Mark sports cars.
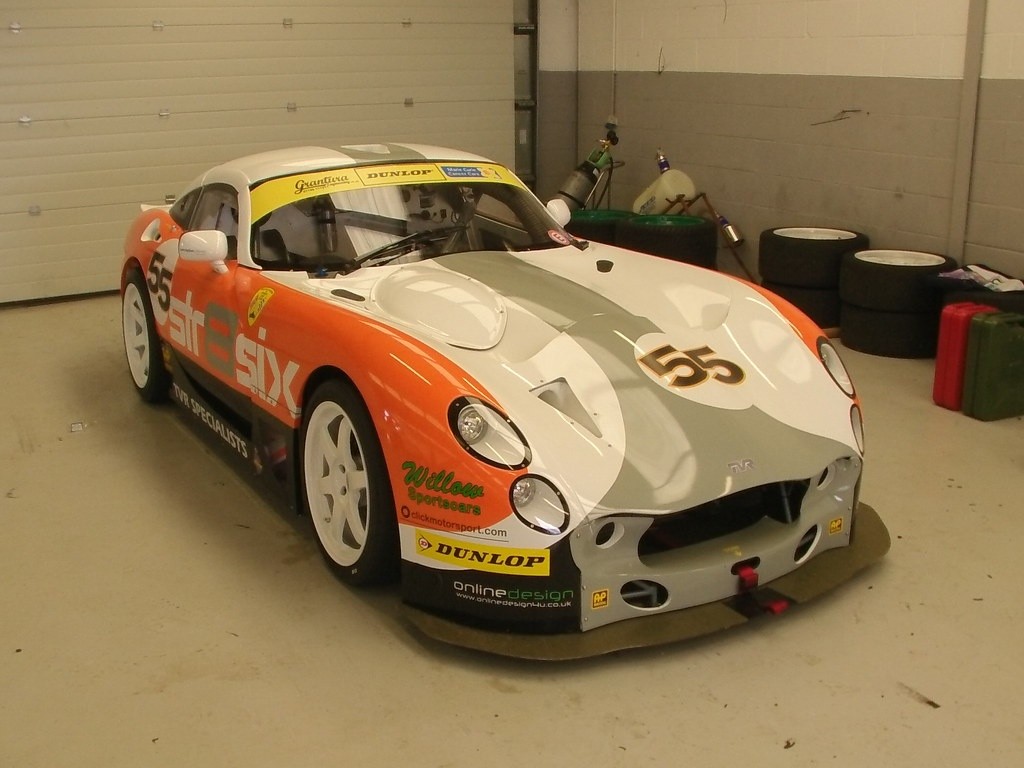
[121,143,892,661]
